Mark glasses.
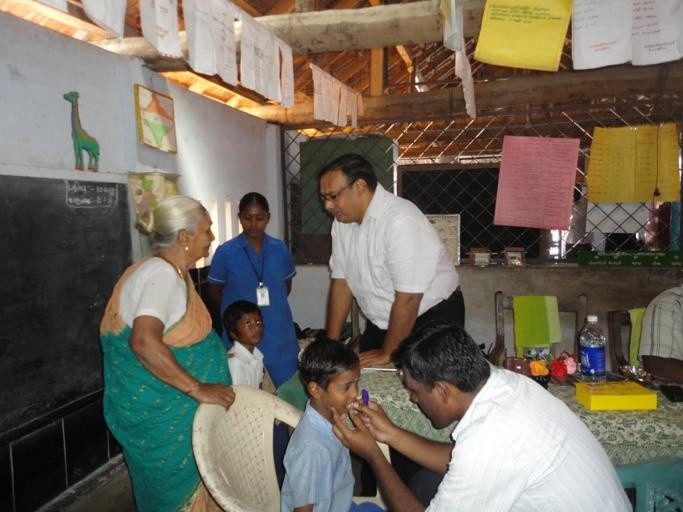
[320,181,354,203]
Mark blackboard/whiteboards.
[397,164,550,265]
[0,163,140,444]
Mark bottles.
[577,313,607,384]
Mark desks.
[272,368,682,511]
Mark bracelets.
[186,380,199,393]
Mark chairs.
[485,289,645,376]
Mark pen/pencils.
[362,390,370,417]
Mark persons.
[204,193,300,487]
[637,286,683,402]
[224,300,264,389]
[280,337,361,511]
[315,153,464,511]
[329,315,635,512]
[99,196,236,512]
